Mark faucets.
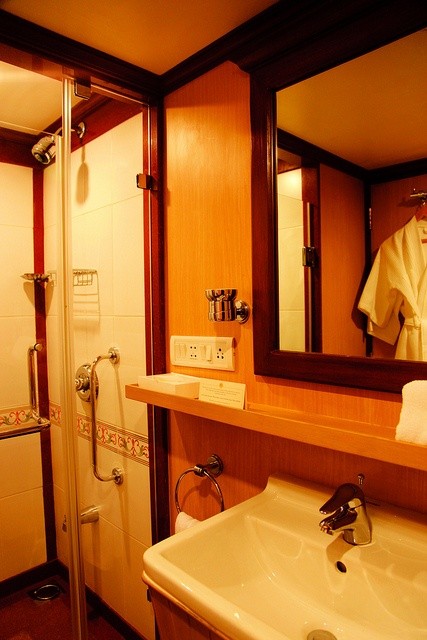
[320,482,372,545]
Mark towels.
[395,380,426,448]
[173,511,201,533]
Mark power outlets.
[169,335,236,373]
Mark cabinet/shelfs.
[143,586,228,639]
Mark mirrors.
[250,16,427,395]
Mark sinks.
[143,472,426,640]
